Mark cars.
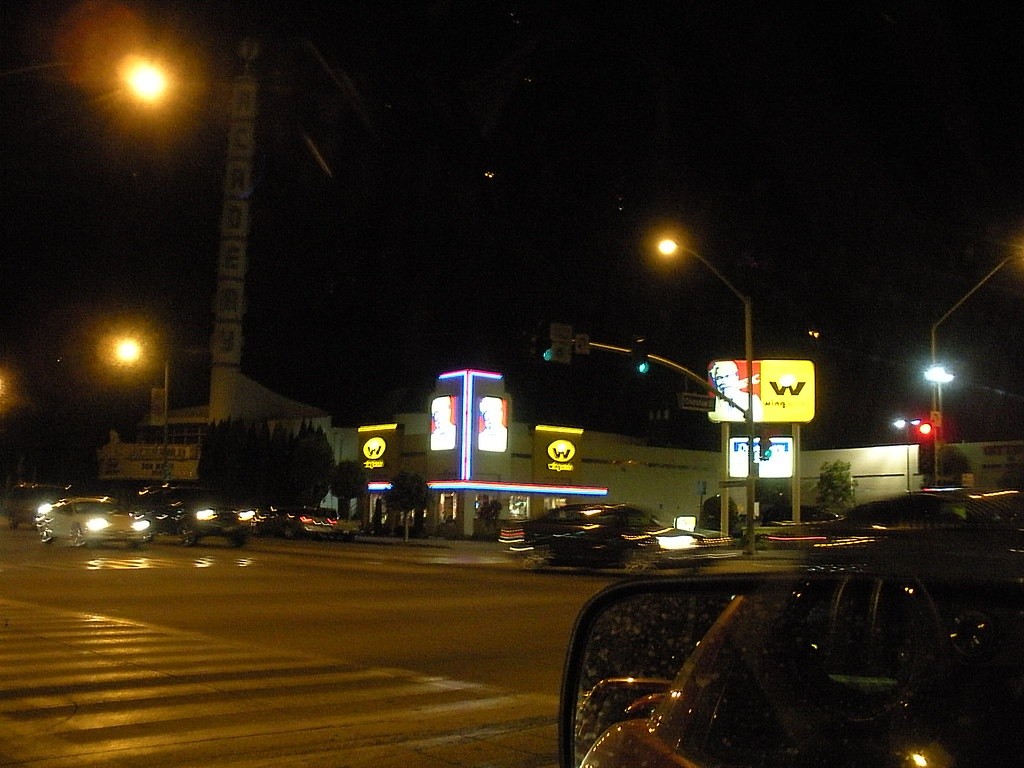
[250,500,361,542]
[576,679,673,767]
[36,498,156,550]
[520,503,728,573]
[808,486,1023,570]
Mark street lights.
[111,338,169,484]
[656,239,755,558]
[893,417,919,493]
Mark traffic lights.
[538,336,551,362]
[635,339,651,376]
[760,429,771,460]
[921,421,932,438]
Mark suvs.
[3,483,79,527]
[132,481,255,550]
